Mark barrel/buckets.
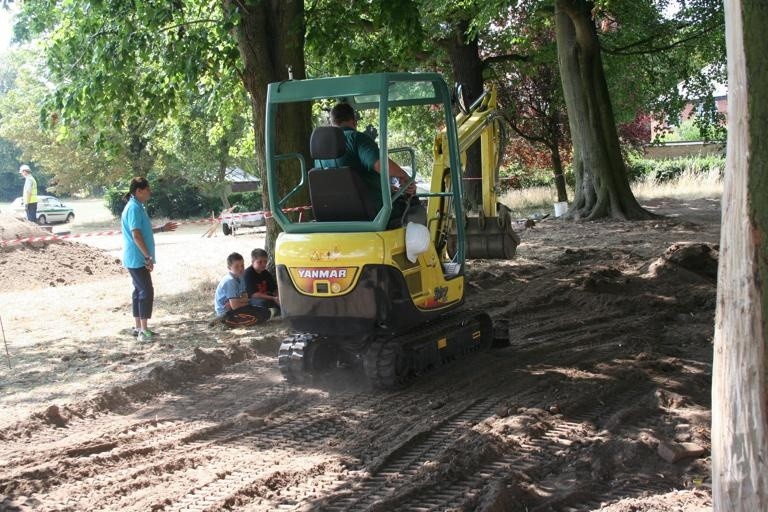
[553,202,568,217]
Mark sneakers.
[208,317,222,329]
[132,327,159,338]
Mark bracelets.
[161,226,165,232]
[145,255,152,261]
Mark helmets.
[405,221,430,263]
[19,165,30,174]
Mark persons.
[245,247,282,318]
[120,177,179,340]
[207,251,273,332]
[19,163,38,224]
[313,102,430,230]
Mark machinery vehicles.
[264,71,520,391]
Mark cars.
[9,194,75,226]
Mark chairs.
[308,126,408,231]
